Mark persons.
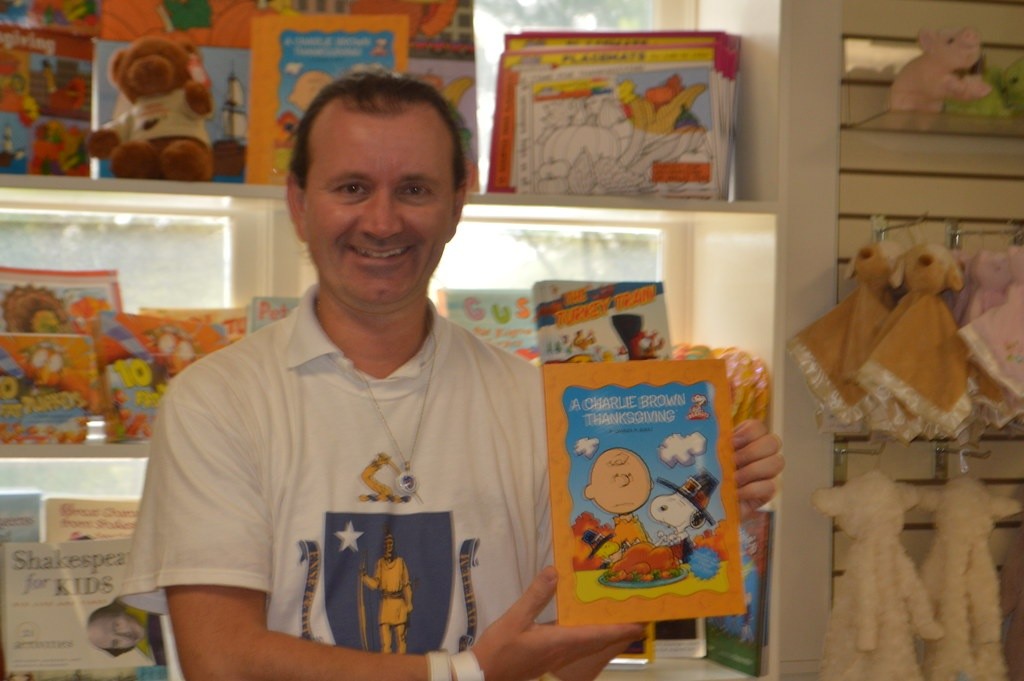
[120,70,786,680]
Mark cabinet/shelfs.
[2,0,1024,681]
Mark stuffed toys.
[915,474,1023,681]
[813,470,944,681]
[884,19,989,123]
[996,52,1023,112]
[939,57,1014,131]
[82,29,221,180]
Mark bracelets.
[423,635,455,680]
[453,650,487,680]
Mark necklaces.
[316,281,448,498]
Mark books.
[250,292,307,333]
[2,265,126,340]
[258,1,481,201]
[38,496,152,542]
[484,17,754,205]
[98,306,226,442]
[536,279,674,363]
[537,352,748,625]
[2,1,97,40]
[0,331,98,450]
[0,483,44,541]
[1,15,89,179]
[88,1,257,182]
[246,11,412,188]
[438,284,540,364]
[2,544,171,680]
[138,305,250,349]
